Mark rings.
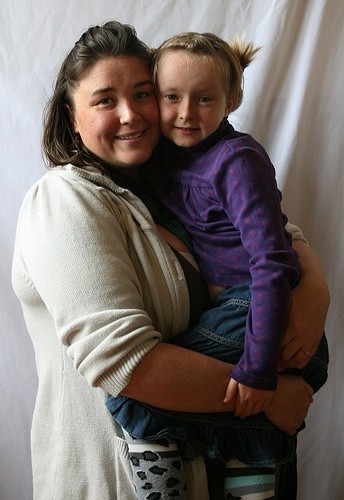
[299,348,312,358]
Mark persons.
[12,23,331,500]
[104,31,329,500]
